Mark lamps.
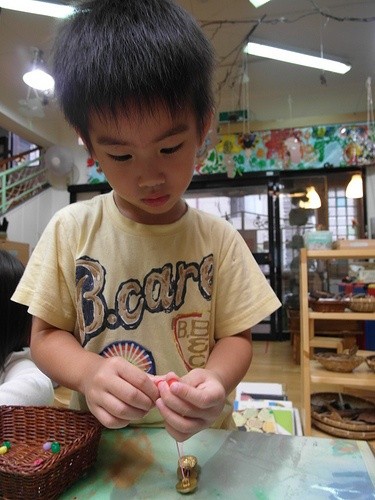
[0,0,75,20]
[242,16,352,75]
[22,51,55,91]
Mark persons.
[10,0,282,443]
[0,248,54,406]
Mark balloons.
[46,144,81,190]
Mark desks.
[57,428,375,500]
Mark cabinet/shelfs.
[298,248,375,454]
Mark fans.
[44,144,75,175]
